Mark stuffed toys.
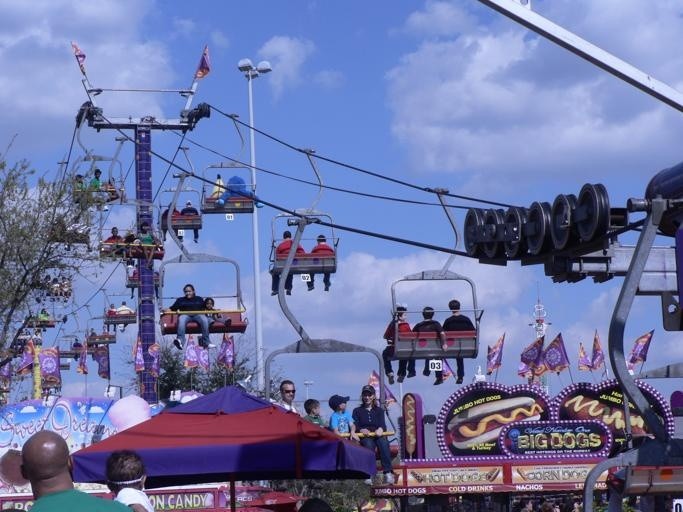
[211,174,263,209]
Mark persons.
[307,235,333,291]
[18,430,137,510]
[271,231,305,295]
[105,448,160,511]
[106,301,133,332]
[383,300,475,385]
[295,480,586,512]
[103,223,162,298]
[14,274,70,348]
[163,201,200,242]
[167,284,232,349]
[277,380,394,481]
[72,167,119,212]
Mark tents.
[68,387,375,488]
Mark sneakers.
[388,372,463,385]
[207,344,217,349]
[383,471,392,483]
[174,339,183,350]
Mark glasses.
[342,402,345,404]
[285,389,295,394]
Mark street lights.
[304,381,314,400]
[238,57,273,399]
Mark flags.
[368,371,396,406]
[4,333,235,388]
[191,45,214,79]
[483,329,654,385]
[70,34,91,77]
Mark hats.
[329,395,350,410]
[362,385,375,393]
